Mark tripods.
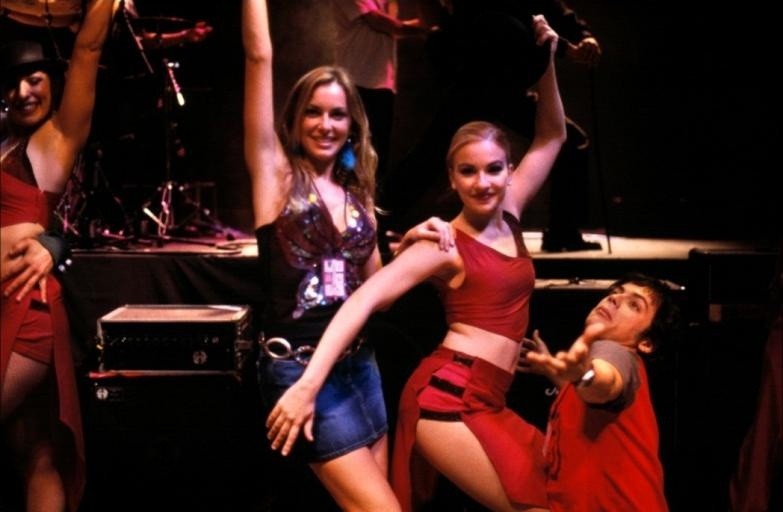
[59,47,238,250]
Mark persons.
[114,0,214,245]
[377,0,604,250]
[240,0,458,511]
[262,10,570,512]
[292,0,445,244]
[0,0,123,510]
[0,229,76,305]
[522,271,683,511]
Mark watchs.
[568,360,597,391]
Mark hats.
[0,38,68,77]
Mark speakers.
[505,277,676,501]
[688,248,783,512]
